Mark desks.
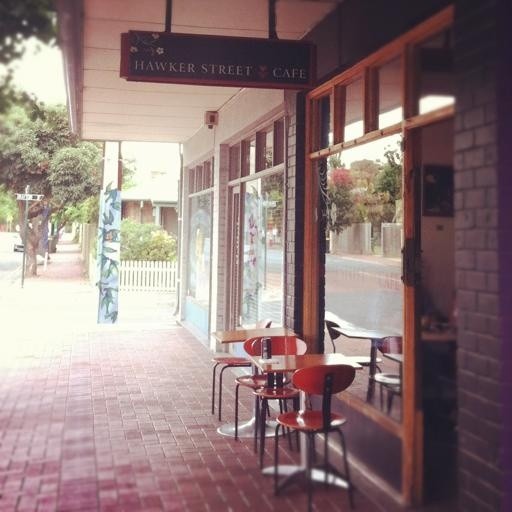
[213,329,301,438]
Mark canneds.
[260,338,272,359]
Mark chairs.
[325,320,402,415]
[234,334,360,512]
[213,321,272,423]
